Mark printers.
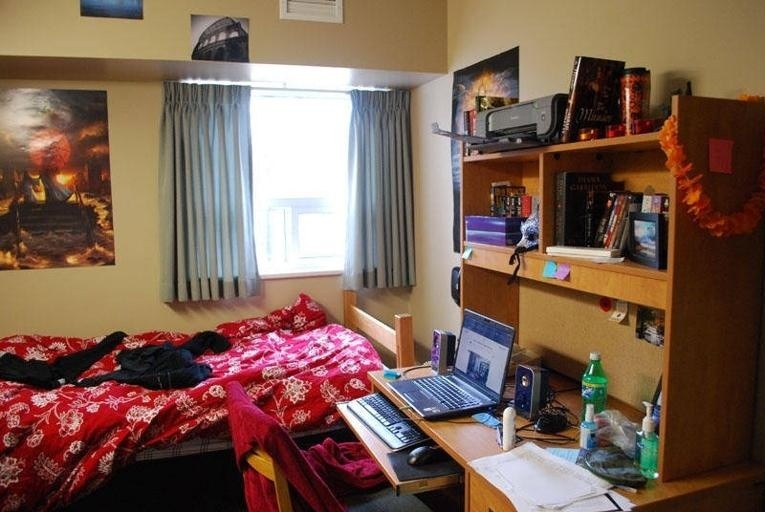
[432,94,567,153]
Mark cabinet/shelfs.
[335,94,764,510]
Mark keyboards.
[348,392,432,451]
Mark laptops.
[386,309,515,421]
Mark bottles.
[581,351,609,423]
[579,402,601,452]
[617,66,653,137]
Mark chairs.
[224,379,443,510]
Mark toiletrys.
[636,401,659,480]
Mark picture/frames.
[630,211,665,269]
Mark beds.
[1,288,414,512]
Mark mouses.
[408,445,437,466]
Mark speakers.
[432,329,456,375]
[515,365,550,420]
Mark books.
[490,180,532,218]
[546,172,670,257]
[559,56,625,143]
